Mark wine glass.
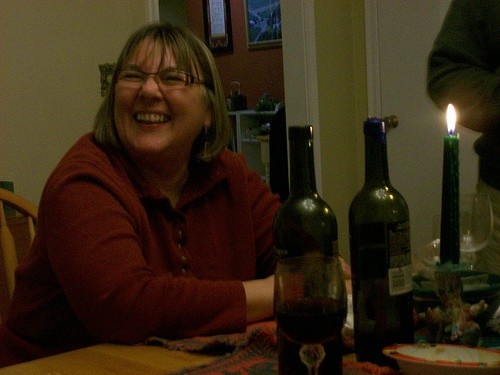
[459,193,493,269]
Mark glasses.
[116,67,211,90]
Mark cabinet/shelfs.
[229,102,283,172]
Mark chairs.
[0,186,38,296]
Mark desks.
[0,322,403,375]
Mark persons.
[0,22,351,370]
[425,0,500,276]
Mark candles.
[440,103,461,266]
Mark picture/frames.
[202,0,233,53]
[244,0,284,52]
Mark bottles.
[348,117,411,363]
[273,124,344,375]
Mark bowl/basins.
[381,341,500,375]
[413,266,500,334]
